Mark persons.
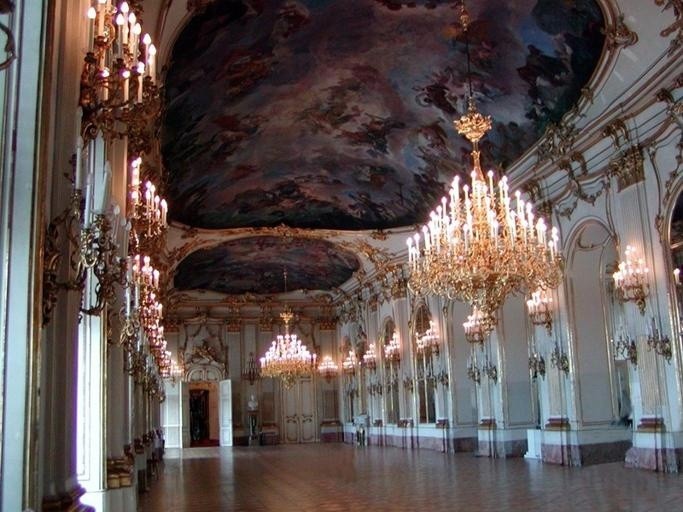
[248,394,258,435]
